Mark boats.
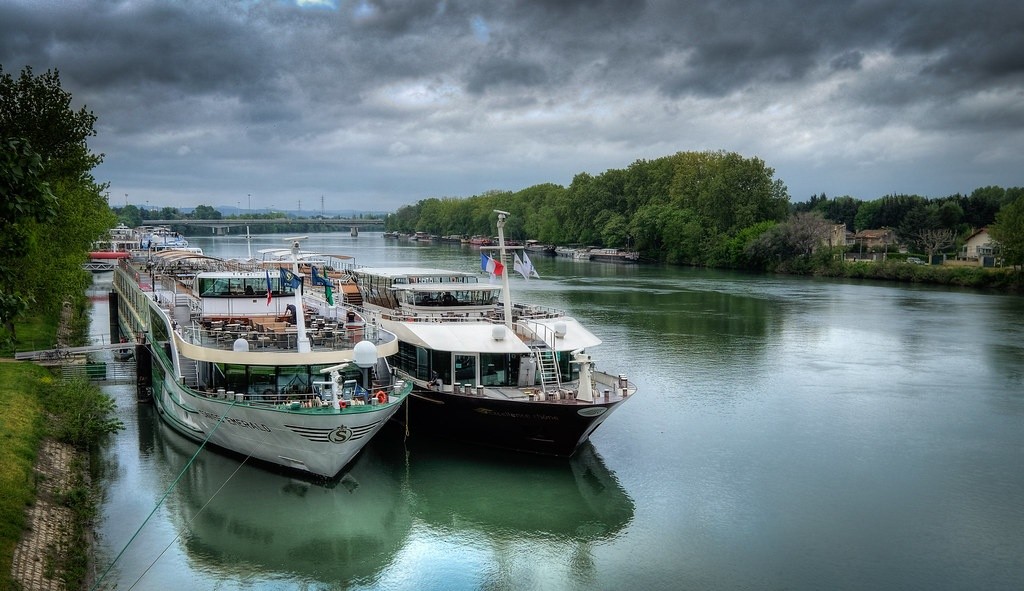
[553,244,590,262]
[591,246,632,260]
[86,223,204,266]
[524,238,550,253]
[353,204,637,470]
[468,237,491,245]
[410,231,432,243]
[382,232,400,239]
[113,245,413,479]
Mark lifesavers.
[375,390,386,404]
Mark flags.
[312,266,335,306]
[514,251,540,281]
[280,268,301,289]
[481,253,504,283]
[266,270,271,305]
[355,384,369,397]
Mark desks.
[147,261,209,286]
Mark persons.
[284,304,296,323]
[220,285,231,296]
[178,261,182,270]
[426,370,443,393]
[456,356,473,368]
[148,240,151,248]
[347,306,356,323]
[140,239,143,249]
[172,230,181,237]
[119,337,129,353]
[243,285,254,295]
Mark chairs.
[203,307,351,348]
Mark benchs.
[258,323,291,334]
[268,328,296,342]
[255,320,287,330]
[267,326,294,334]
[248,317,276,329]
